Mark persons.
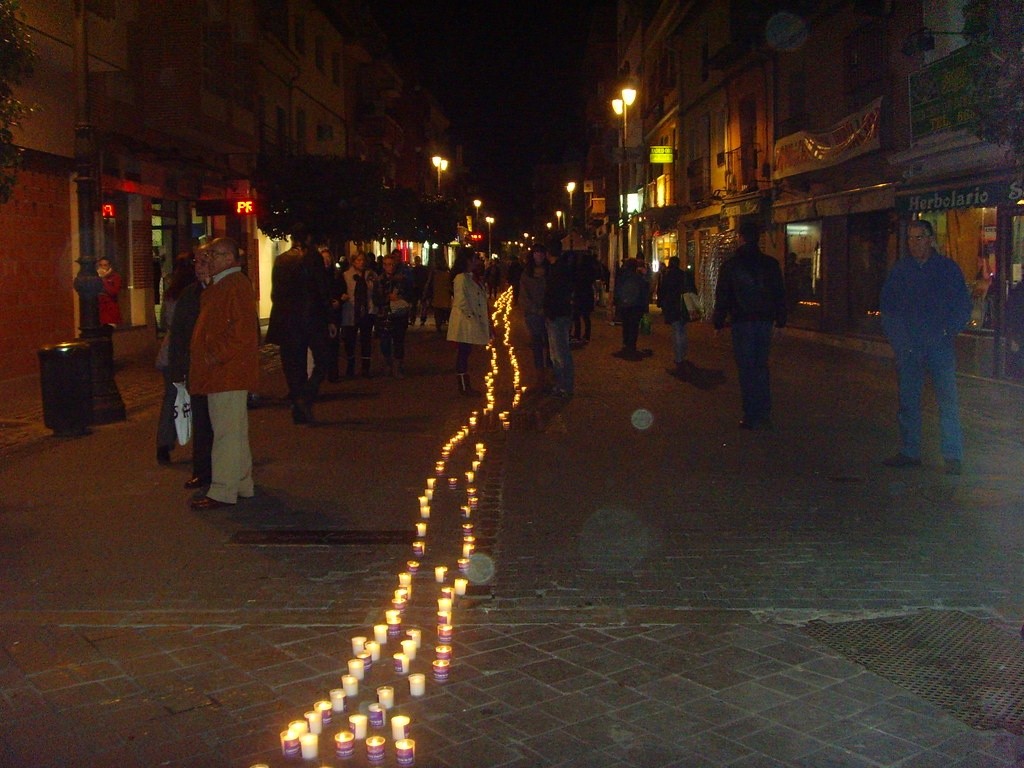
[256,221,338,425]
[878,218,973,477]
[710,221,789,432]
[294,237,699,405]
[151,236,277,513]
[1006,264,1024,380]
[784,252,803,310]
[96,256,123,357]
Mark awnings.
[629,177,1024,227]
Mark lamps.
[739,179,771,195]
[710,189,728,202]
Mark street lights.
[485,214,493,268]
[609,61,638,259]
[566,181,576,254]
[472,196,482,255]
[428,141,448,242]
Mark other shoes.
[883,452,920,468]
[944,460,961,476]
[192,497,234,510]
[186,475,205,488]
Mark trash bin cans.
[37,342,97,437]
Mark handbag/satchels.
[247,343,289,398]
[172,377,193,447]
[683,291,703,322]
[390,286,413,314]
[639,317,652,335]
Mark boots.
[457,372,481,399]
